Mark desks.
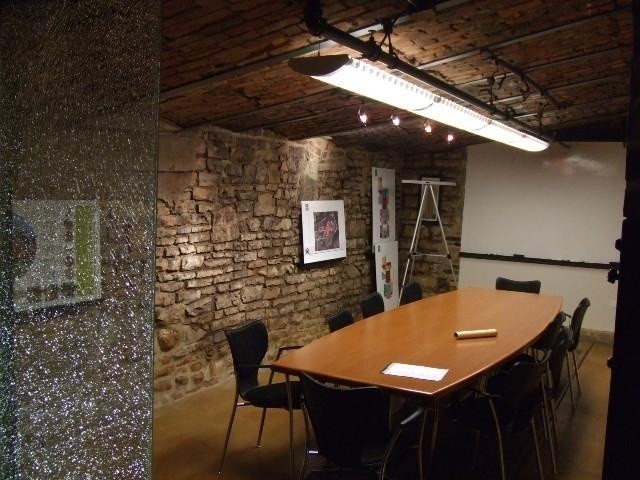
[270,286,563,477]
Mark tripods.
[396,185,457,306]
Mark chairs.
[298,372,429,480]
[358,293,385,320]
[218,321,306,475]
[326,310,353,333]
[430,277,591,480]
[398,282,422,306]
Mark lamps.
[288,2,554,154]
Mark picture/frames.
[299,199,348,264]
